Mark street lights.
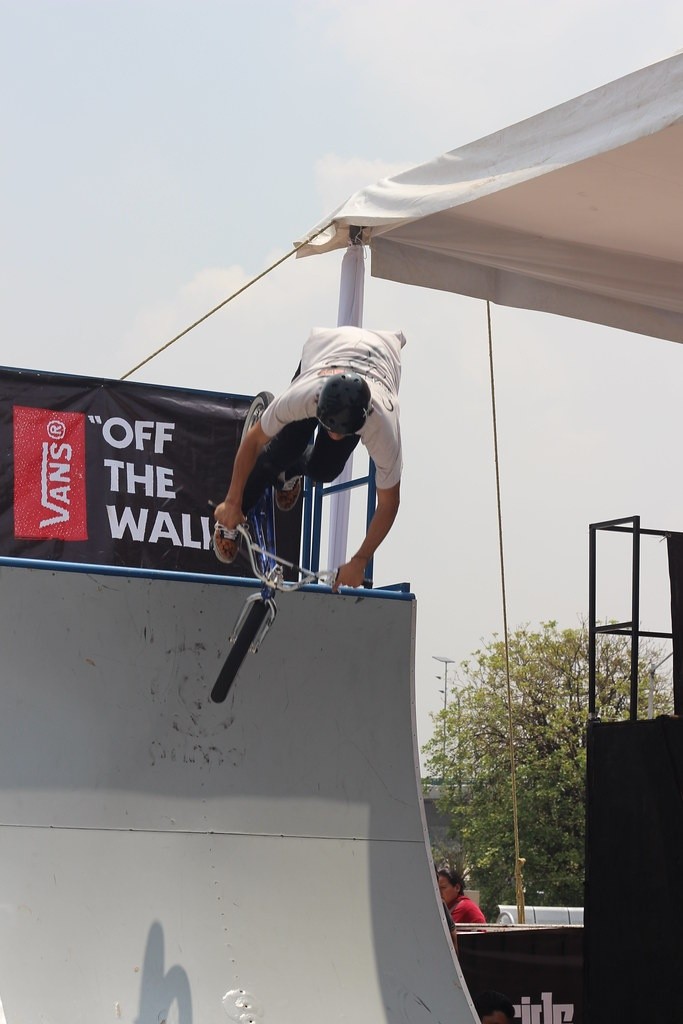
[430,653,456,783]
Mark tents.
[295,57,683,582]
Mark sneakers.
[212,515,248,564]
[274,474,302,512]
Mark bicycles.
[201,392,376,704]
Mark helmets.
[316,372,371,435]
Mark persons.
[434,868,515,1024]
[213,327,405,590]
